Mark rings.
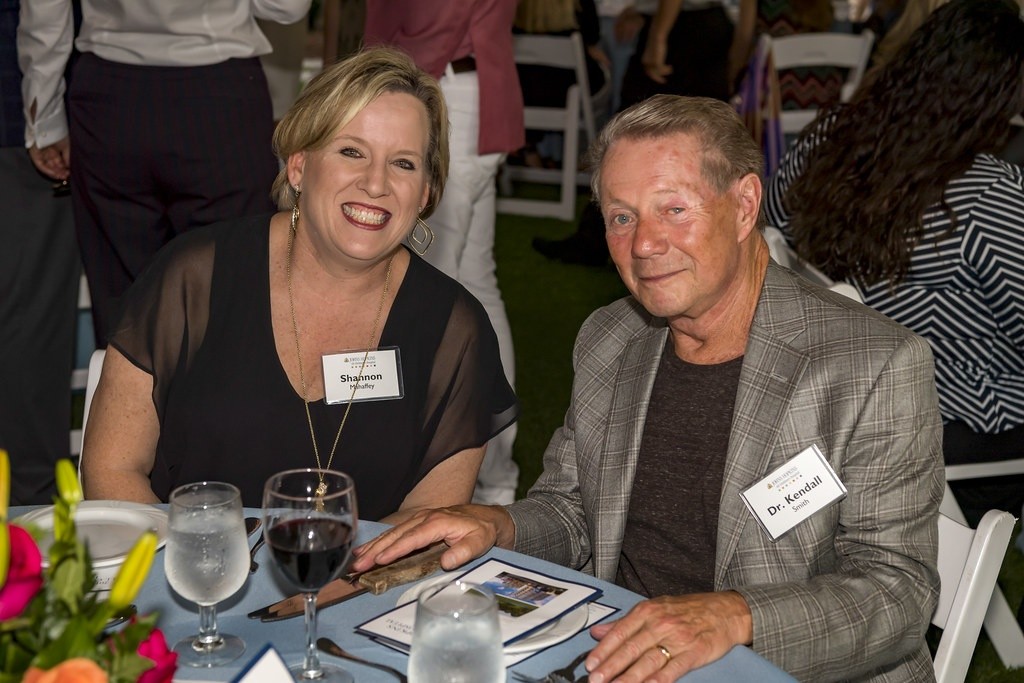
[658,645,674,660]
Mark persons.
[355,0,529,509]
[753,0,1023,547]
[0,0,85,515]
[86,43,492,531]
[13,0,319,351]
[346,90,948,683]
[514,1,965,270]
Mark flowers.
[1,447,181,683]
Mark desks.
[6,504,796,683]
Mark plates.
[13,498,170,568]
[395,570,589,653]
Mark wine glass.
[261,468,355,683]
[163,480,251,668]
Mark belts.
[443,55,477,76]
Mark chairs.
[493,32,599,220]
[762,225,1024,683]
[746,30,877,148]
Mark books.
[353,556,603,655]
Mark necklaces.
[285,205,394,515]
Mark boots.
[531,203,608,268]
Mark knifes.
[247,541,453,622]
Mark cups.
[406,580,508,683]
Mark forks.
[242,515,280,572]
[512,650,597,683]
